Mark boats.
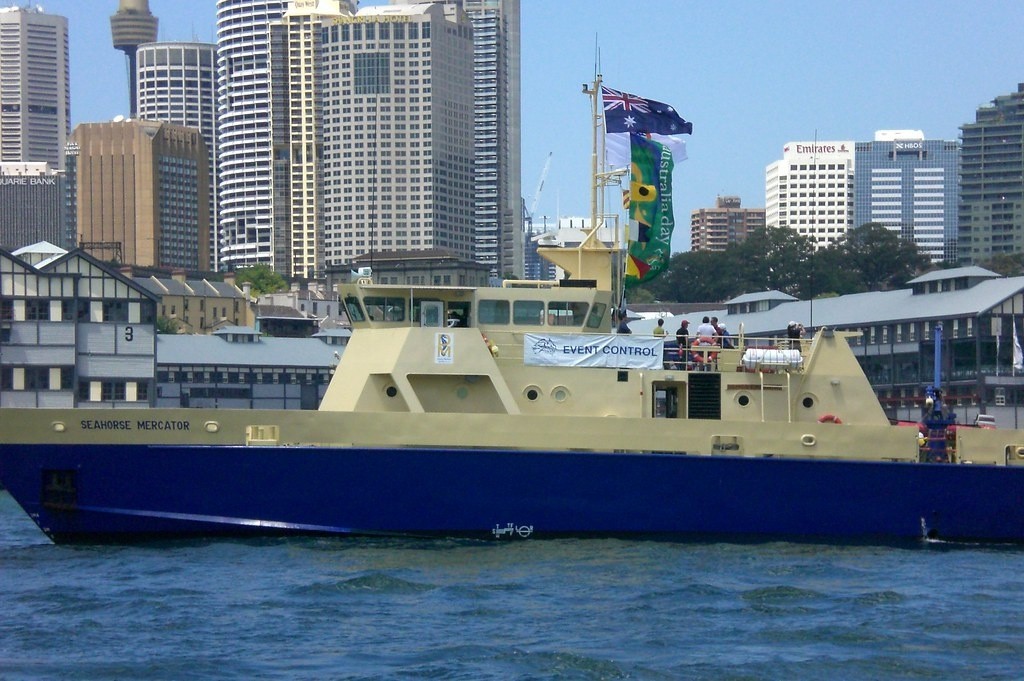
[1,41,1024,550]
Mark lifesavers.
[691,337,719,362]
[817,413,842,424]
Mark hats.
[682,320,690,325]
[718,323,726,329]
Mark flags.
[604,132,689,289]
[1013,316,1023,371]
[601,84,693,135]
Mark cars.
[974,414,996,426]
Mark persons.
[618,312,632,334]
[570,303,602,329]
[695,316,734,371]
[788,321,806,355]
[677,320,691,370]
[653,318,669,339]
